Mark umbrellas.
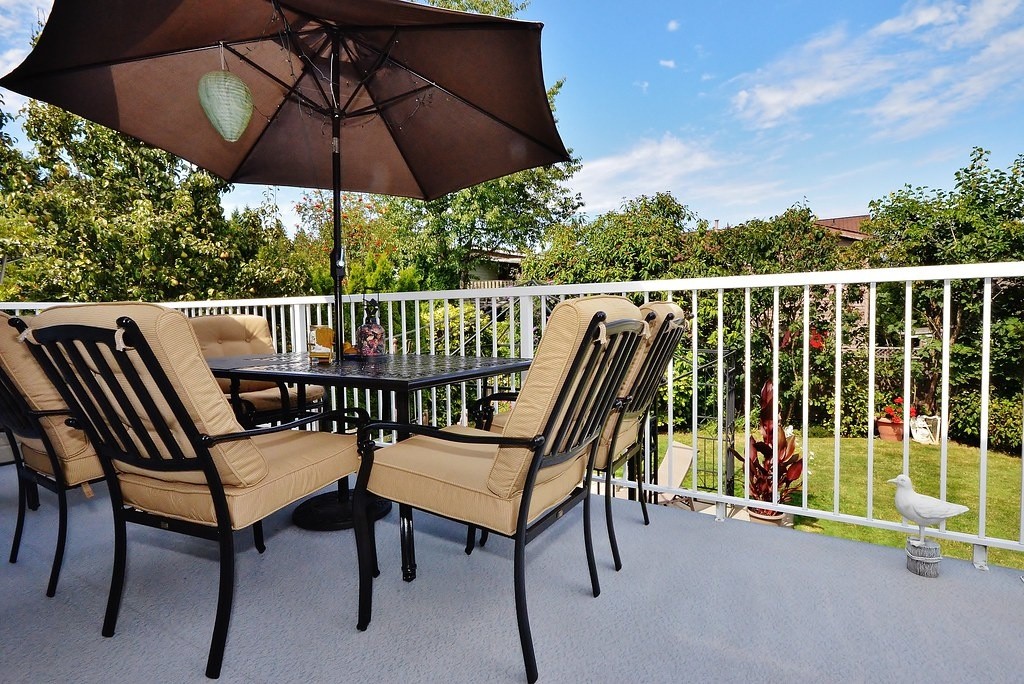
[0,0,571,368]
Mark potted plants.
[728,379,803,526]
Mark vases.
[875,414,904,442]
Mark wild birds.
[884,473,971,549]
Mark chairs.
[188,314,324,429]
[349,296,645,684]
[13,300,380,679]
[480,301,685,572]
[0,310,119,597]
[646,441,702,512]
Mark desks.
[206,352,532,583]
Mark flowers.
[882,397,916,424]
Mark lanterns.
[198,71,254,142]
